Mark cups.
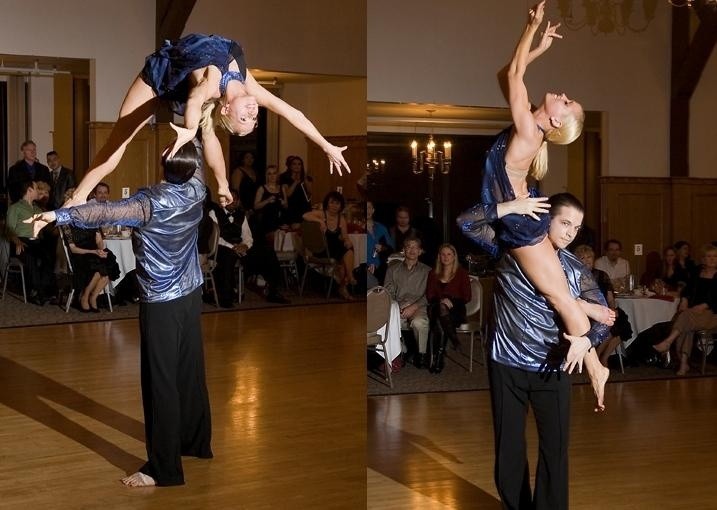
[122,230,130,239]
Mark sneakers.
[414,356,428,369]
[265,289,288,303]
[76,297,89,314]
[90,300,101,314]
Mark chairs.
[367,285,396,389]
[298,221,338,305]
[202,223,221,310]
[1,257,28,305]
[57,225,113,315]
[429,276,486,373]
[614,345,629,378]
[279,262,300,293]
[696,329,717,377]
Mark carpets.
[0,290,366,331]
[369,330,717,396]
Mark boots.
[440,314,465,353]
[434,330,449,373]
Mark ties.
[50,172,59,201]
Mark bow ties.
[227,211,236,219]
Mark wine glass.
[117,225,121,237]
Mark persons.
[61,187,121,313]
[57,32,351,208]
[574,244,632,368]
[367,201,394,292]
[675,241,692,288]
[197,185,213,269]
[209,188,291,310]
[457,193,610,510]
[33,180,51,208]
[427,243,472,375]
[5,179,62,305]
[251,164,288,236]
[8,139,52,204]
[280,155,313,223]
[658,247,683,295]
[302,190,357,303]
[650,244,716,377]
[477,0,610,413]
[383,237,428,369]
[593,240,630,288]
[389,208,417,253]
[23,113,215,488]
[230,147,260,211]
[47,150,76,209]
[86,182,112,207]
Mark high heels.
[652,342,671,353]
[676,367,691,377]
[337,289,354,300]
[345,276,356,285]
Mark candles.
[443,139,452,160]
[410,139,419,158]
[426,134,436,159]
